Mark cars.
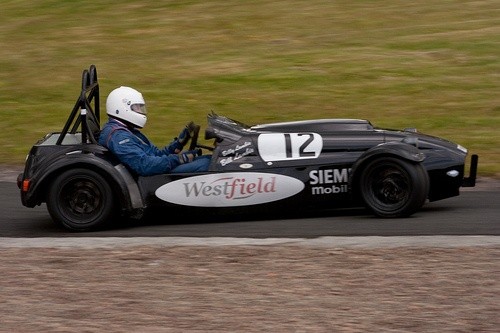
[17,63,478,233]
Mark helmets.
[106,86,147,129]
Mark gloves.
[175,121,200,150]
[180,148,202,164]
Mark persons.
[97,86,212,177]
